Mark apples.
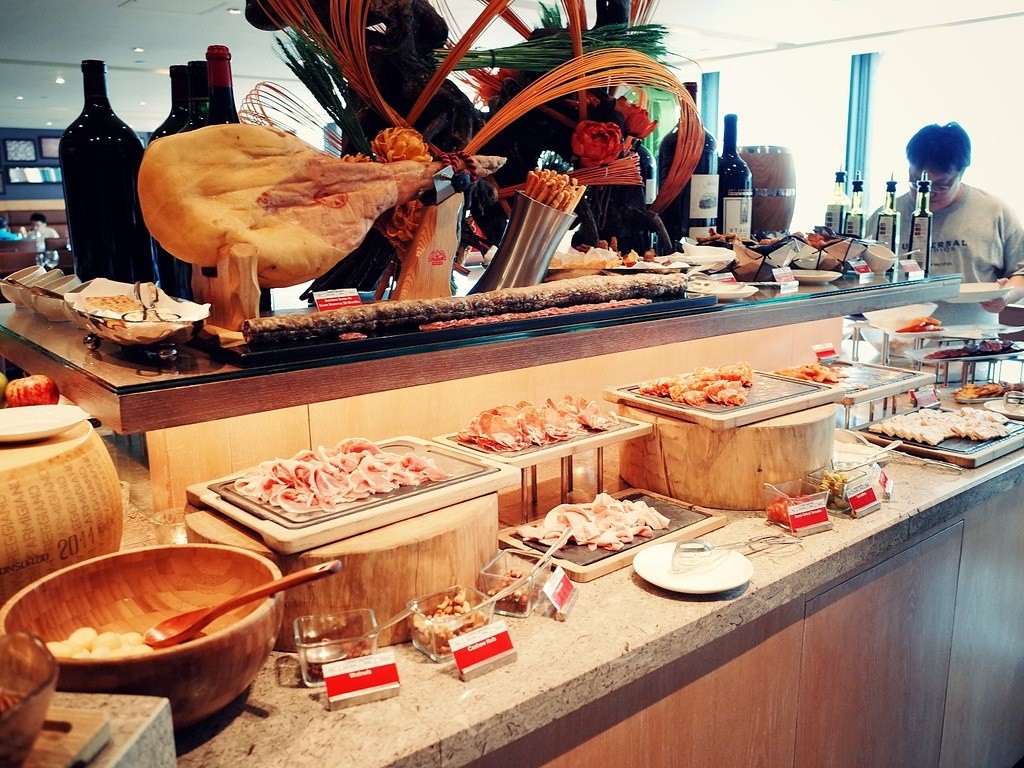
[0,369,59,409]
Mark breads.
[86,295,145,313]
[956,381,1024,400]
[868,407,1010,446]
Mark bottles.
[718,114,753,243]
[905,170,933,279]
[824,170,852,233]
[842,170,866,240]
[875,172,902,275]
[58,59,159,287]
[147,45,272,329]
[657,82,720,257]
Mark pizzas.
[773,364,840,383]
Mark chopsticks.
[524,166,587,214]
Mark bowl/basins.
[0,265,96,329]
[859,302,939,357]
[405,582,496,663]
[480,548,552,619]
[0,630,60,768]
[761,478,830,530]
[861,244,896,275]
[293,607,380,687]
[806,465,868,516]
[0,543,284,728]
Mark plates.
[0,404,91,441]
[632,541,753,595]
[685,280,759,301]
[942,282,1014,304]
[984,400,1024,420]
[791,269,843,285]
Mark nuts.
[413,589,488,656]
[486,569,541,614]
[819,469,852,510]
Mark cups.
[465,190,578,296]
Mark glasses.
[908,168,960,193]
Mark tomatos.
[768,493,816,526]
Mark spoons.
[141,558,343,648]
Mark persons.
[0,212,62,270]
[864,122,1024,344]
